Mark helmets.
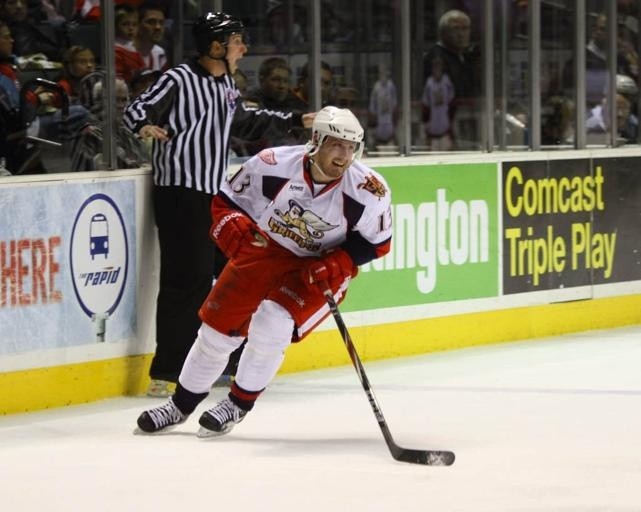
[304,105,367,161]
[616,74,641,104]
[192,10,246,51]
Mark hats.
[619,16,640,34]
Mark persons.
[0,0,641,179]
[131,104,394,434]
[121,9,318,400]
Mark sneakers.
[213,374,236,387]
[199,395,249,432]
[137,395,192,433]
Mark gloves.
[210,210,279,268]
[301,248,359,295]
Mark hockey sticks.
[318,277,456,463]
